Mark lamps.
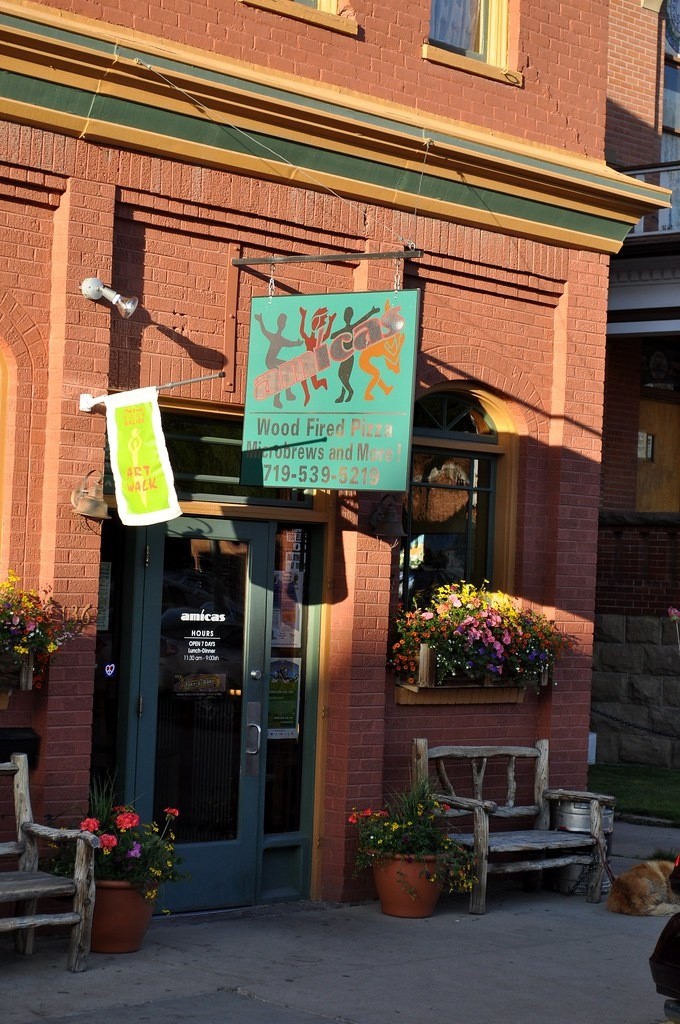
[71,468,112,519]
[79,277,138,319]
[638,430,655,463]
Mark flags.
[103,385,183,527]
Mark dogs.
[604,860,680,917]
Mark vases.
[90,880,153,954]
[370,849,448,918]
[0,678,10,710]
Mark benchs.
[0,752,99,972]
[412,737,615,914]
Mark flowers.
[53,788,193,919]
[349,775,480,899]
[391,580,571,696]
[0,569,73,688]
